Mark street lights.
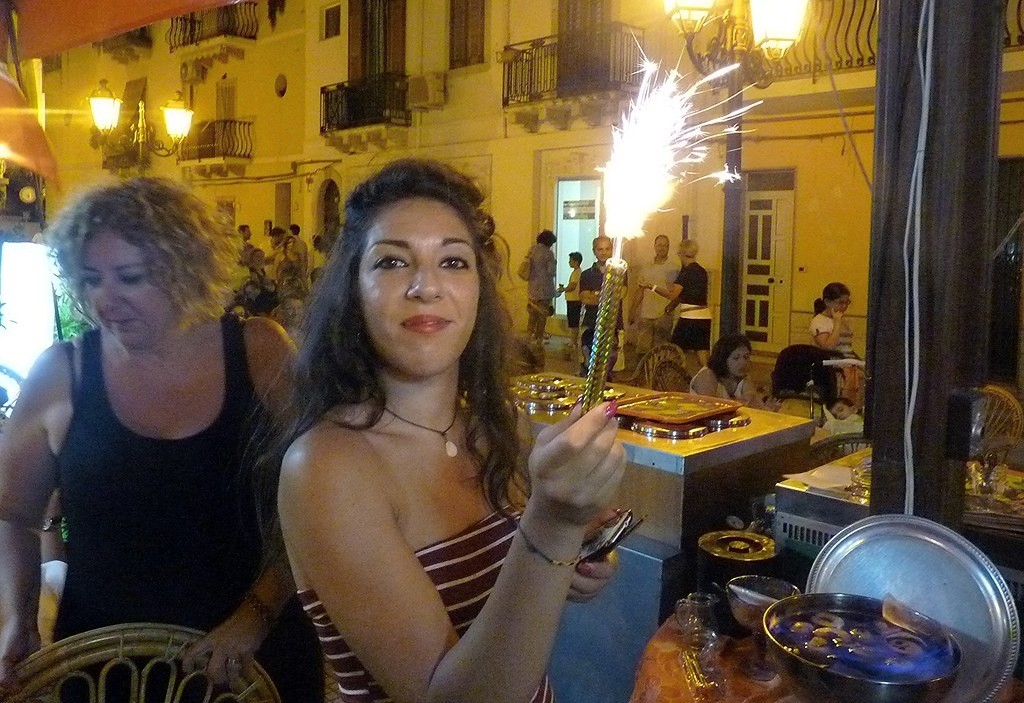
[86,79,194,179]
[662,0,813,339]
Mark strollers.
[810,358,866,434]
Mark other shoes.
[562,341,578,348]
[528,330,550,345]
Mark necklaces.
[385,406,460,458]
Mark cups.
[675,591,721,650]
[971,461,1009,507]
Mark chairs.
[627,343,685,390]
[967,385,1023,465]
[0,622,282,703]
[809,431,872,466]
[652,360,692,392]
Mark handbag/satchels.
[517,244,538,281]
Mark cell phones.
[573,509,634,566]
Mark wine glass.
[725,575,801,681]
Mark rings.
[226,658,239,664]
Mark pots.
[763,592,964,703]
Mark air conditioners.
[406,73,445,109]
[179,59,203,82]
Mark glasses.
[832,298,852,308]
[676,252,682,257]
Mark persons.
[0,177,325,703]
[822,398,864,432]
[810,281,855,357]
[519,229,713,372]
[278,156,627,703]
[689,334,782,413]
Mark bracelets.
[516,525,580,568]
[244,591,275,626]
[42,515,62,532]
